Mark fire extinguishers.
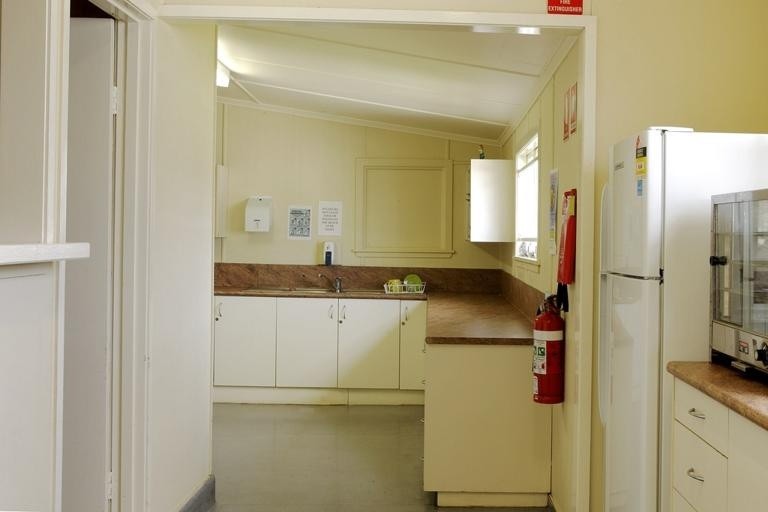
[533,295,565,405]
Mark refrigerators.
[597,126,768,512]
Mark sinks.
[295,288,328,294]
[242,286,292,293]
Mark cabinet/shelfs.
[671,377,767,512]
[464,160,516,246]
[400,299,552,508]
[214,296,401,406]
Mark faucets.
[318,273,341,293]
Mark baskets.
[384,280,426,295]
